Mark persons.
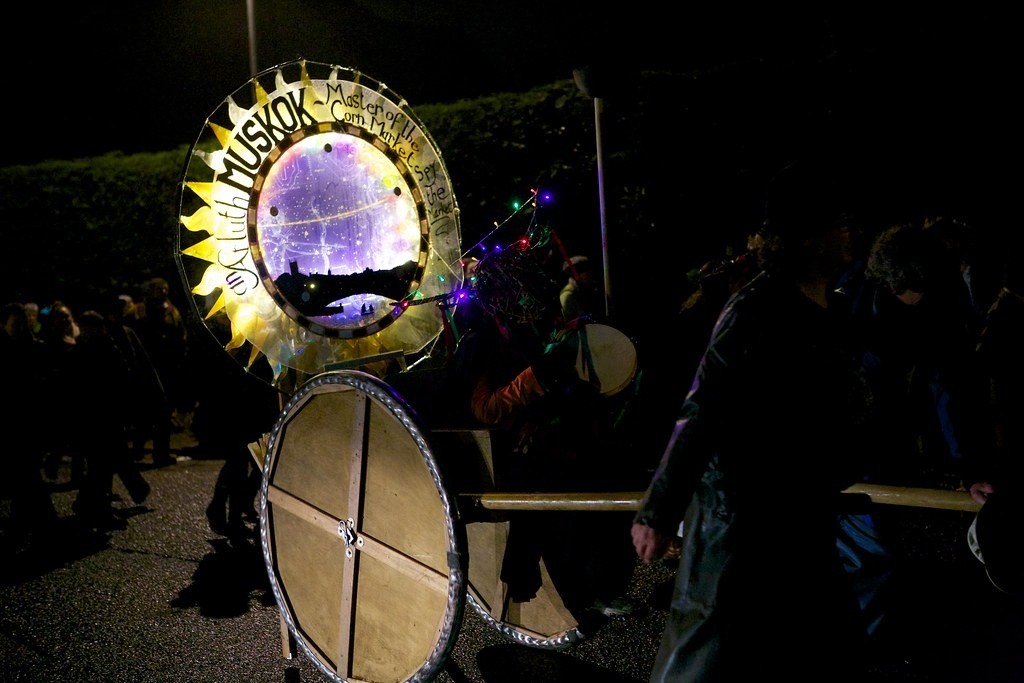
[0,219,1024,683]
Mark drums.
[575,323,639,397]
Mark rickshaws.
[258,352,987,683]
